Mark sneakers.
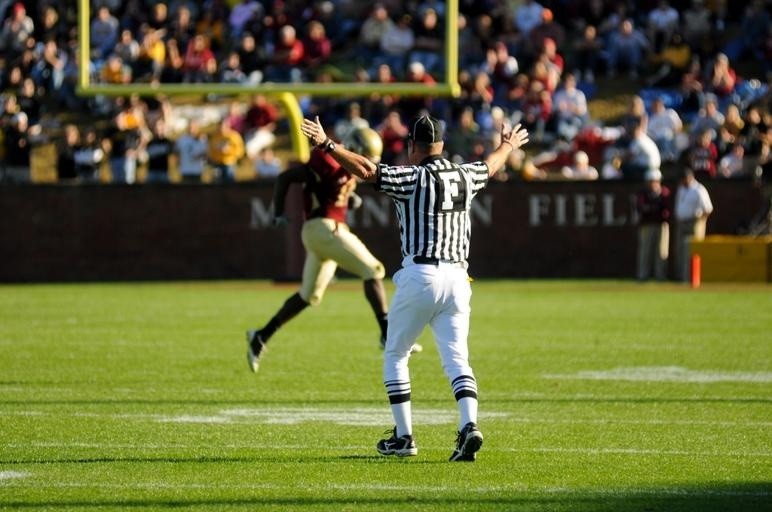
[449,422,484,462]
[379,335,423,353]
[246,328,264,373]
[376,426,418,457]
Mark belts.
[413,256,438,265]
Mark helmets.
[339,127,383,163]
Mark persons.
[0,0,772,182]
[635,170,672,281]
[300,111,530,462]
[247,127,422,372]
[672,167,720,281]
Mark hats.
[407,114,443,143]
